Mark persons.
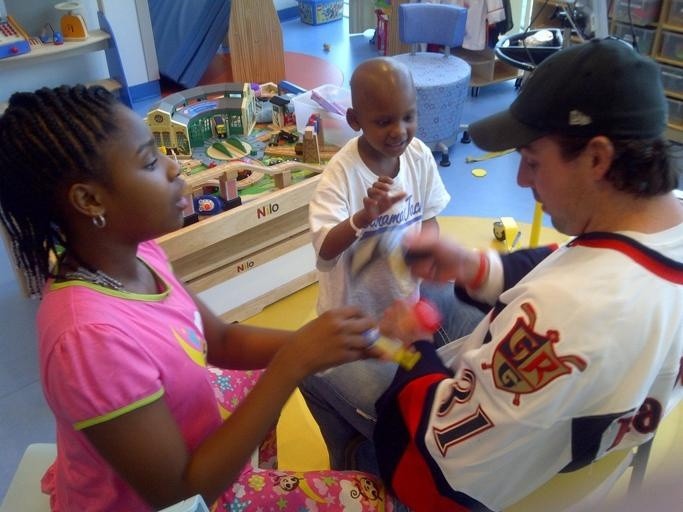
[0,85,394,512]
[309,56,451,328]
[295,35,683,512]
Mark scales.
[54,2,89,41]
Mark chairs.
[389,2,471,167]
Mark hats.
[468,35,669,153]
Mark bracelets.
[349,214,363,237]
[467,249,485,290]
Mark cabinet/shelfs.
[604,0,683,147]
[0,10,133,118]
